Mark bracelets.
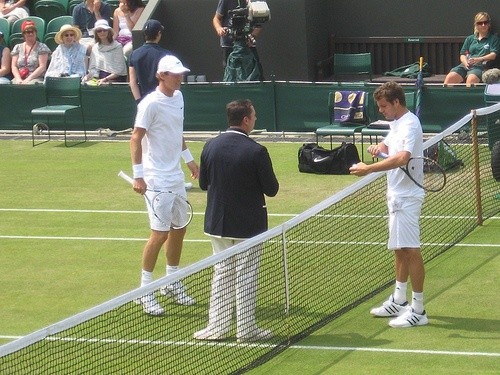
[181,148,194,164]
[135,98,141,105]
[132,164,144,179]
[105,77,108,81]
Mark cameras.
[224,0,270,47]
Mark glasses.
[26,29,36,33]
[65,34,75,37]
[97,29,108,32]
[477,20,490,25]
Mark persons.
[113,0,145,57]
[213,0,262,82]
[11,20,52,85]
[0,0,29,34]
[73,0,114,37]
[349,81,426,328]
[193,98,279,340]
[130,55,200,315]
[129,20,174,104]
[443,10,500,88]
[84,19,127,85]
[43,24,88,85]
[0,32,12,84]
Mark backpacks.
[384,61,431,79]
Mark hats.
[157,55,190,74]
[88,19,114,38]
[21,20,35,31]
[142,20,164,32]
[55,24,82,44]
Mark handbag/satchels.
[298,142,363,174]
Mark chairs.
[10,38,25,49]
[361,92,415,162]
[315,90,374,162]
[44,37,59,53]
[47,15,74,31]
[33,0,65,16]
[10,31,23,40]
[0,18,10,45]
[11,16,45,44]
[43,33,57,42]
[332,52,373,81]
[34,5,60,23]
[67,0,122,13]
[30,76,87,146]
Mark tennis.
[352,163,357,167]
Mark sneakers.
[237,329,274,343]
[194,326,225,340]
[160,282,195,306]
[371,295,410,317]
[134,294,164,315]
[388,307,428,328]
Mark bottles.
[466,51,475,70]
